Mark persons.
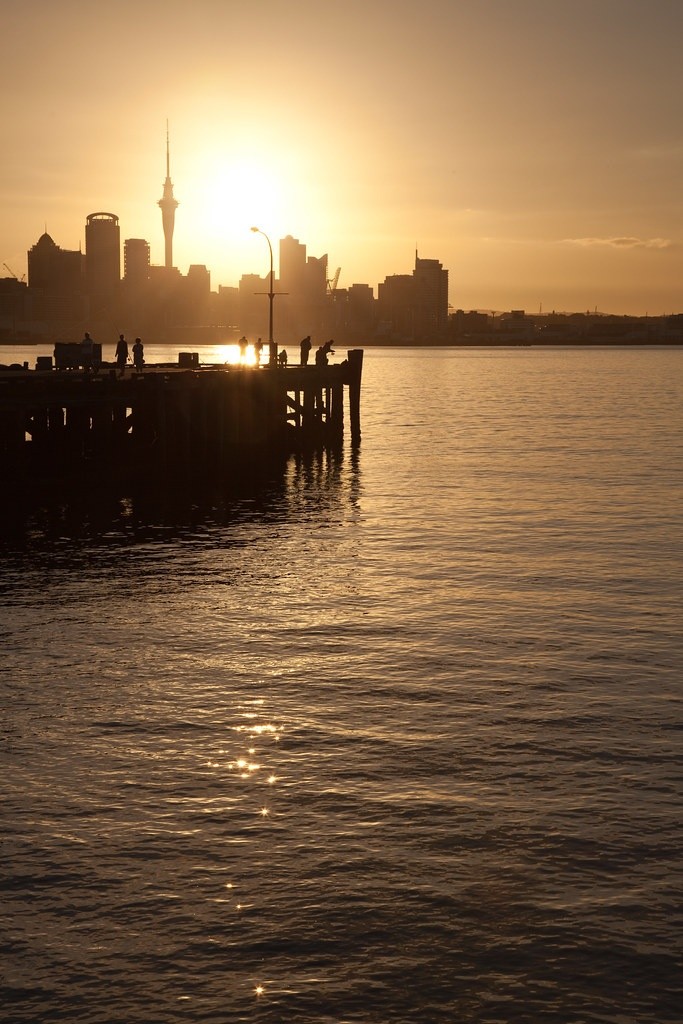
[300,335,312,367]
[80,332,93,374]
[132,337,144,371]
[315,340,337,366]
[253,337,264,366]
[238,335,249,356]
[279,349,288,368]
[115,335,128,376]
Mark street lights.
[250,227,274,369]
